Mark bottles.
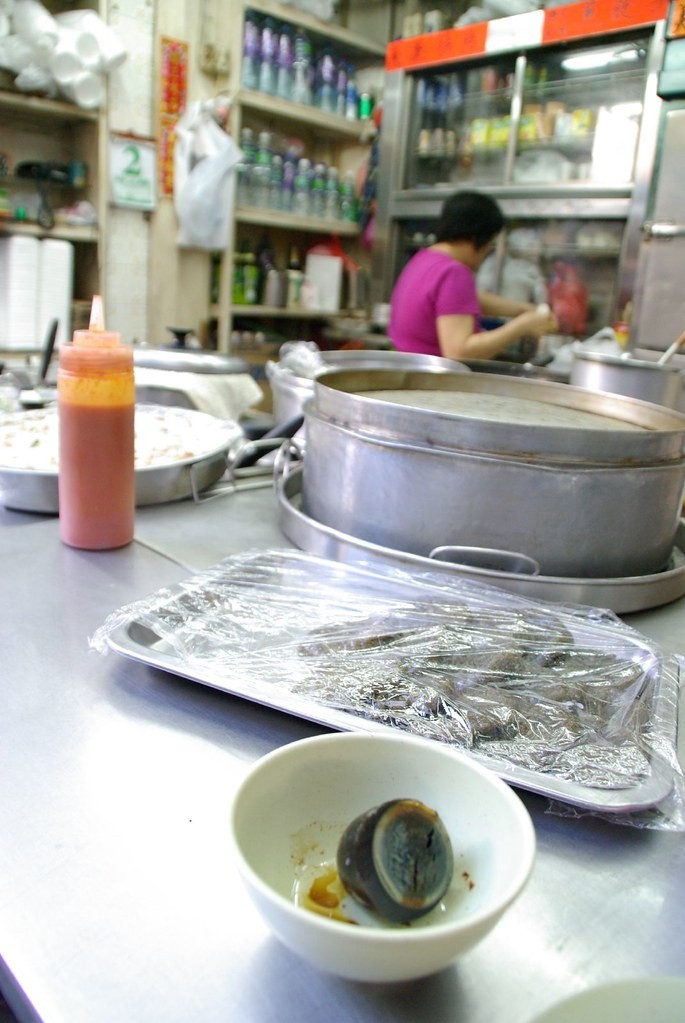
[56,295,136,551]
[208,11,374,307]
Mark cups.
[0,0,128,109]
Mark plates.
[105,551,679,814]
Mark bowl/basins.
[225,732,538,987]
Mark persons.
[475,226,545,337]
[387,191,551,360]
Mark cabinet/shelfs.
[197,0,387,356]
[0,0,110,355]
[383,18,669,339]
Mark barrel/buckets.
[568,342,685,416]
[271,368,685,577]
